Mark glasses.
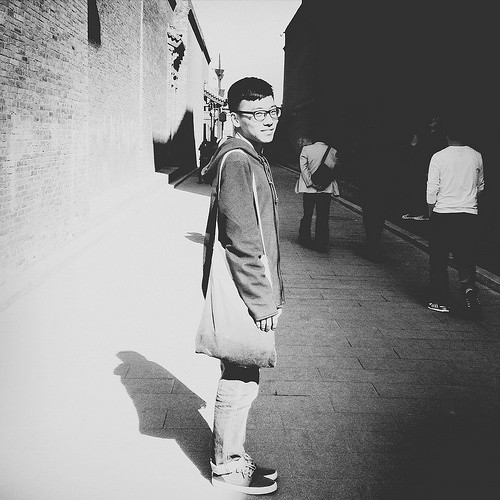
[236,107,282,122]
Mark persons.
[201,76,286,495]
[426,122,484,317]
[298,128,339,252]
[197,136,218,185]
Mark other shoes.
[428,303,449,313]
[465,287,480,308]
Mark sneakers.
[235,455,277,481]
[212,462,277,495]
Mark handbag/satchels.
[311,163,334,190]
[196,240,277,369]
